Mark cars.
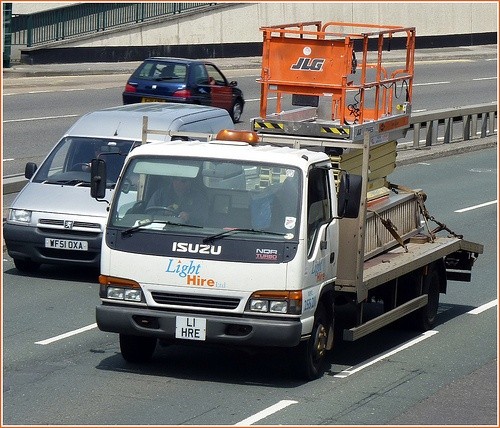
[118,56,246,125]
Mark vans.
[2,102,237,282]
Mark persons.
[74,142,109,177]
[144,175,211,227]
[195,65,215,85]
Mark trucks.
[90,20,487,376]
